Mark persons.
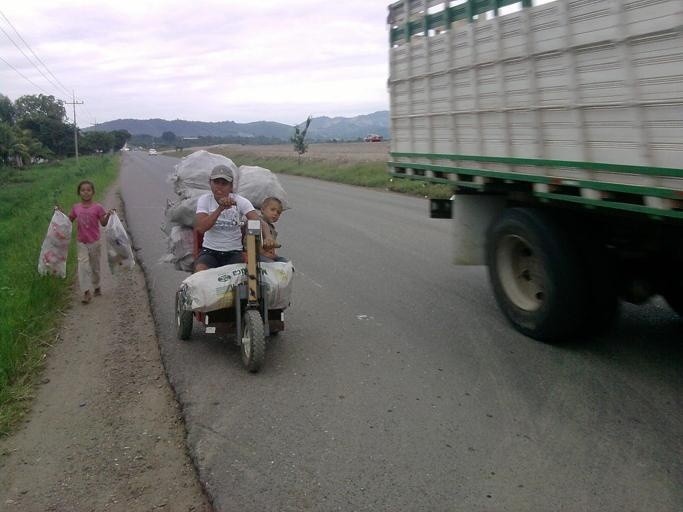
[193,163,281,322]
[253,195,289,264]
[51,179,117,303]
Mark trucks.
[388,0,683,330]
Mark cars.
[364,134,383,142]
[149,148,157,155]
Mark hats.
[209,165,234,182]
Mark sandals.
[81,288,102,303]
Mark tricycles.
[175,218,284,372]
